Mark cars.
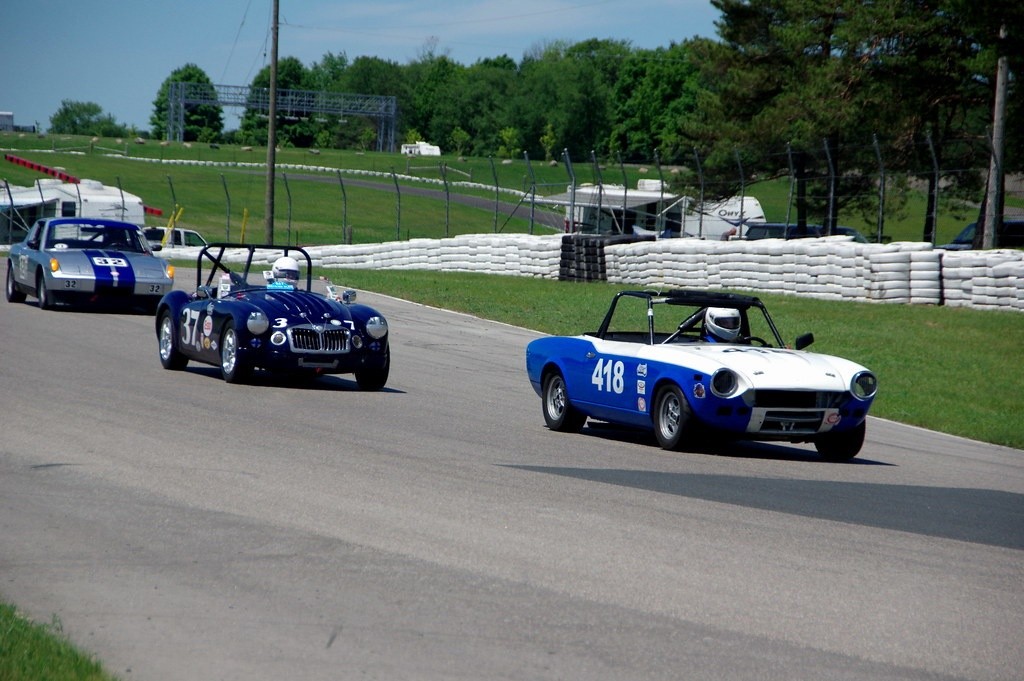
[142,225,208,251]
[935,219,1024,251]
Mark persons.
[698,306,773,347]
[269,256,300,290]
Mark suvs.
[742,224,872,244]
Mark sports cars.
[6,216,173,316]
[155,241,389,392]
[525,287,878,461]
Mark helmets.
[272,257,300,288]
[705,307,741,342]
